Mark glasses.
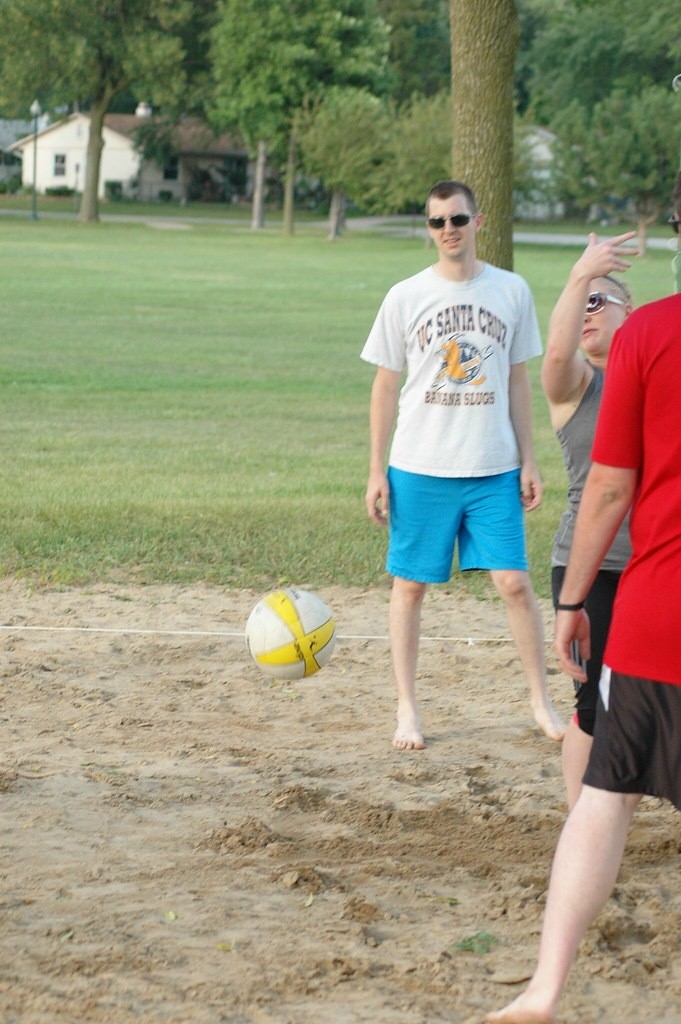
[668,215,681,233]
[426,213,480,229]
[585,291,625,316]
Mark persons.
[541,230,641,810]
[478,169,681,1024]
[358,182,566,754]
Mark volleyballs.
[245,588,337,680]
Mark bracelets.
[556,600,585,611]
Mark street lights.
[29,98,43,220]
